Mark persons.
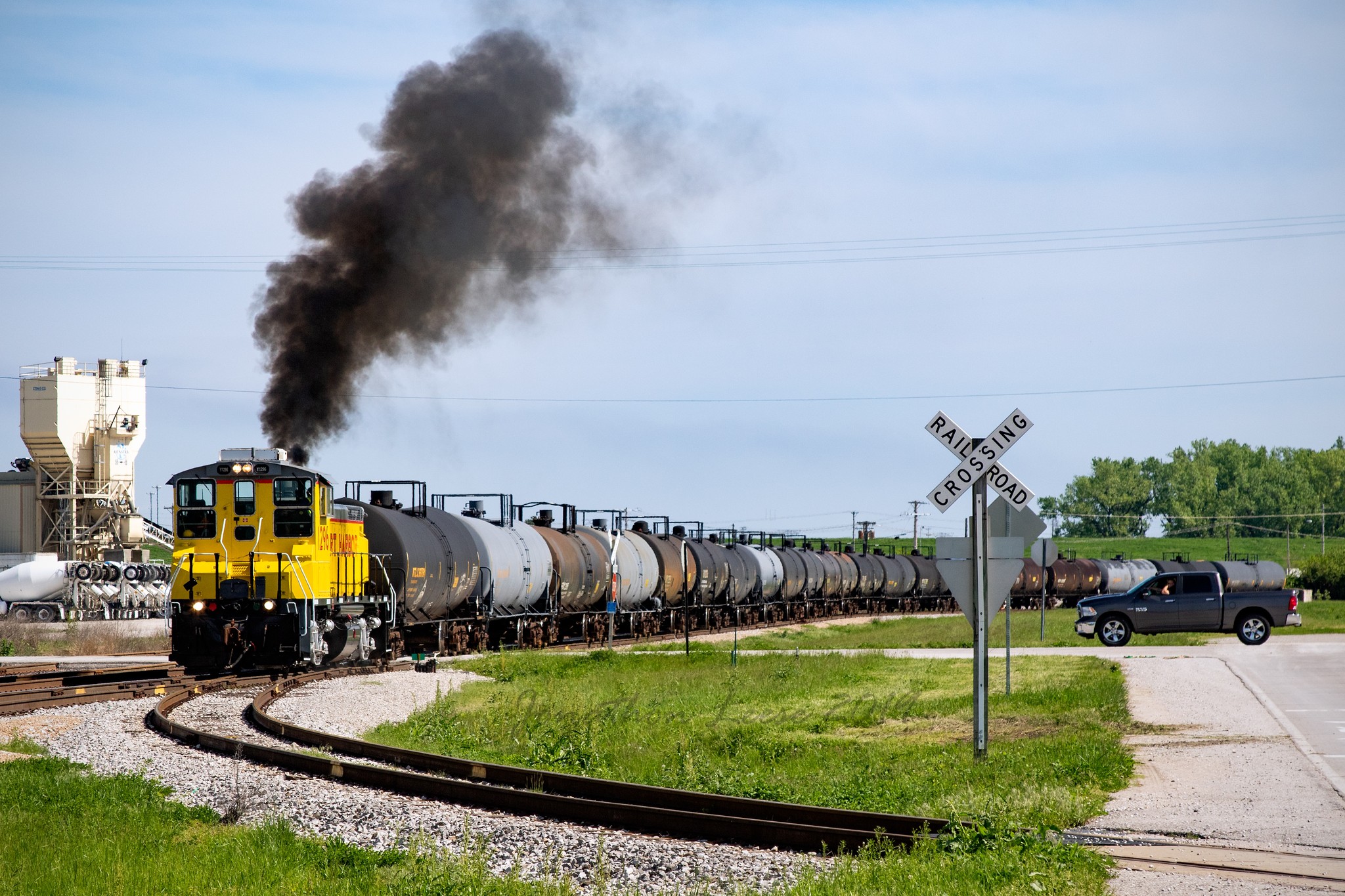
[287,486,313,536]
[1145,578,1176,595]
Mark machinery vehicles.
[0,560,81,623]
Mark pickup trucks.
[1074,571,1303,646]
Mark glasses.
[1166,582,1173,585]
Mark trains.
[163,449,1288,682]
